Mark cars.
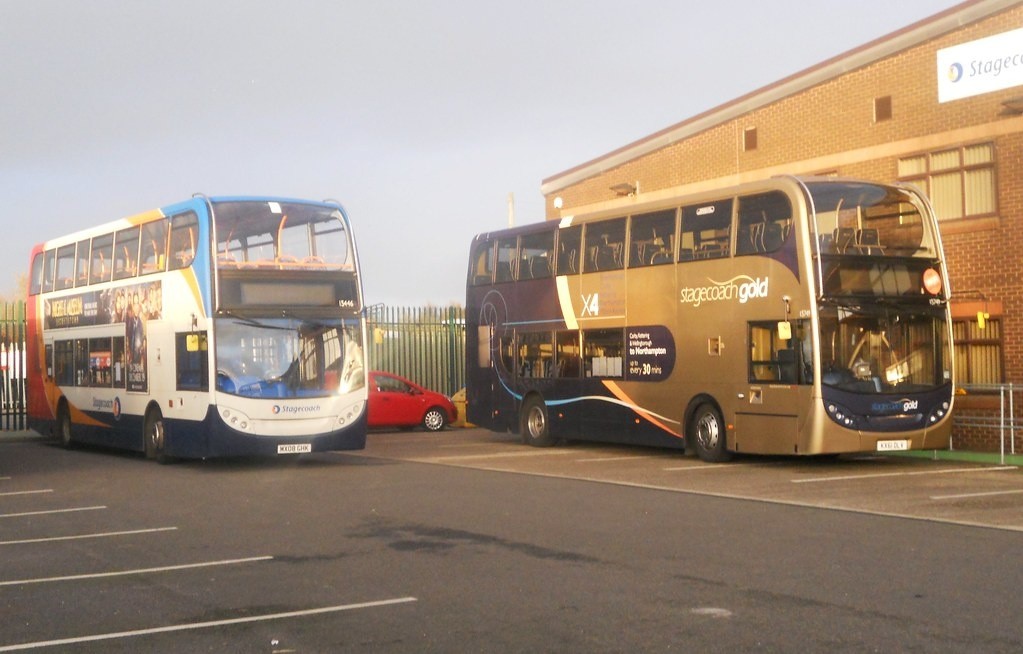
[367,371,459,432]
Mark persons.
[97,281,161,378]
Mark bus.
[25,191,369,463]
[466,173,990,463]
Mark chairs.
[638,241,721,264]
[568,248,591,273]
[820,227,887,255]
[529,256,548,278]
[777,349,806,384]
[593,244,616,270]
[496,261,511,281]
[41,254,194,291]
[617,241,643,268]
[509,258,530,280]
